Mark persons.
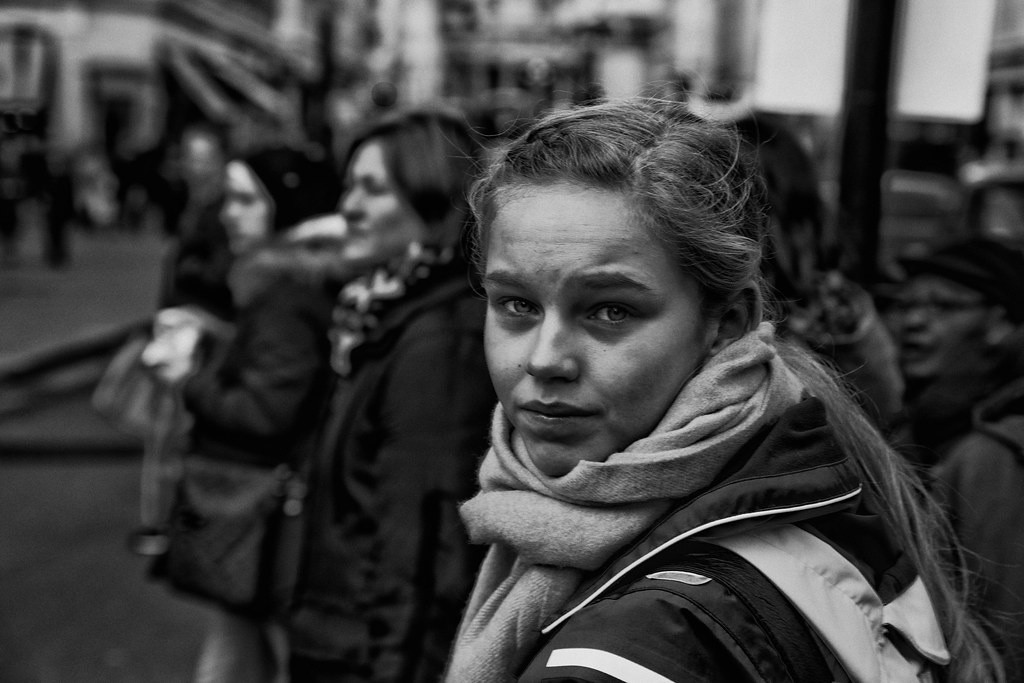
[0,93,227,582]
[445,105,998,683]
[138,144,341,683]
[285,110,501,683]
[702,111,1024,683]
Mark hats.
[895,241,1024,329]
[230,147,339,237]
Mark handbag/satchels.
[88,338,192,445]
[145,460,291,613]
